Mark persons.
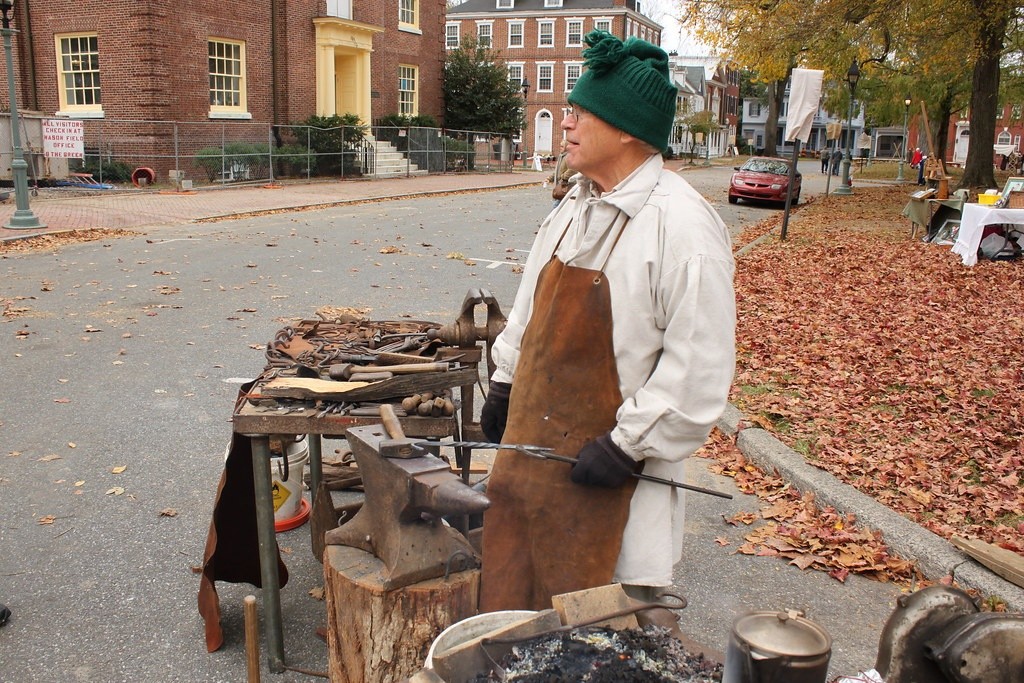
[479,34,735,614]
[544,141,577,212]
[911,148,922,168]
[832,148,842,176]
[801,148,806,157]
[820,146,830,174]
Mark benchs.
[946,161,960,167]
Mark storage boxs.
[927,178,948,199]
[977,195,1001,204]
[911,190,933,202]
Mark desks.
[233,318,457,676]
[912,198,962,244]
[951,203,1024,267]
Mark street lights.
[896,91,912,181]
[521,75,531,167]
[0,0,48,230]
[833,56,860,195]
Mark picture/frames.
[1001,176,1024,197]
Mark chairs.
[930,188,970,243]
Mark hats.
[567,26,678,155]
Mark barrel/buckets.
[225,436,310,532]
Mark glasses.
[566,105,595,122]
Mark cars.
[728,156,802,210]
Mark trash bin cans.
[492,143,502,161]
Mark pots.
[721,609,833,683]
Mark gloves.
[479,380,513,450]
[570,432,643,492]
[561,179,569,188]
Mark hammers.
[379,404,429,458]
[329,362,449,382]
